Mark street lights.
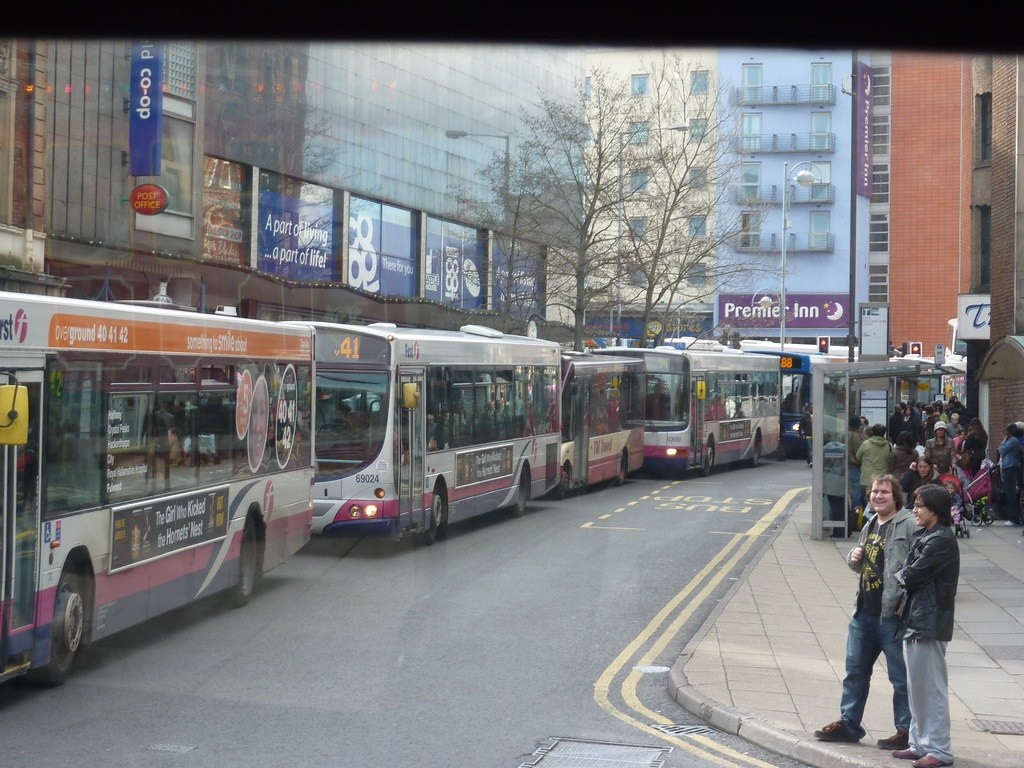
[779,160,823,350]
[616,127,692,340]
[445,129,511,237]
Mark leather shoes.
[912,754,954,768]
[892,748,924,759]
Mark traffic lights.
[816,336,831,355]
[889,340,896,359]
[910,343,924,360]
[897,342,909,358]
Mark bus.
[588,337,782,479]
[554,352,646,491]
[273,318,567,543]
[2,292,317,690]
[738,337,857,456]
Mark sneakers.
[877,727,910,750]
[814,720,860,743]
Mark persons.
[648,382,670,420]
[823,395,1024,538]
[428,393,524,452]
[814,474,925,750]
[733,402,745,418]
[141,400,186,495]
[799,403,813,468]
[892,484,960,768]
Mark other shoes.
[809,462,812,468]
[1004,520,1020,527]
[954,520,960,526]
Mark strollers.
[933,467,972,540]
[960,462,1000,527]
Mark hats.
[849,414,862,427]
[1014,421,1024,431]
[933,421,947,432]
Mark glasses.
[911,502,926,509]
